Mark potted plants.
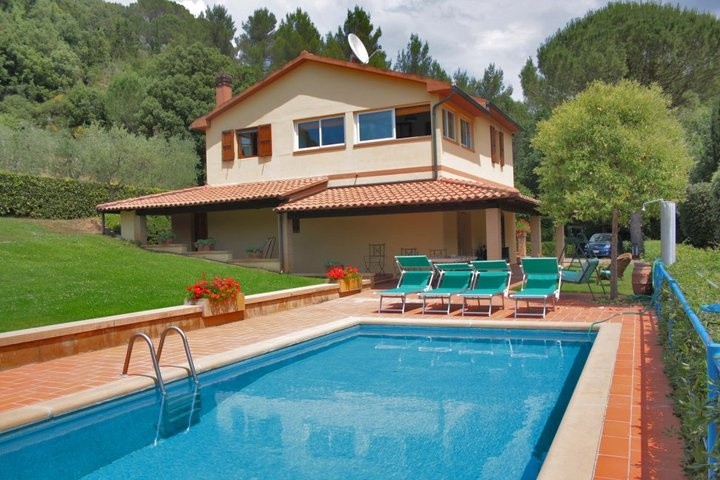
[244,246,264,258]
[158,232,176,244]
[195,239,216,251]
[632,252,653,295]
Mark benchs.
[596,253,631,288]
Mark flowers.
[327,264,364,279]
[184,270,241,308]
[516,218,532,233]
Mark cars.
[575,232,623,259]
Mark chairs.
[374,248,563,319]
[363,242,386,276]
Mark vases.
[516,229,525,238]
[338,278,362,293]
[184,292,245,317]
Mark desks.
[428,256,478,263]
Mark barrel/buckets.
[632,267,653,295]
